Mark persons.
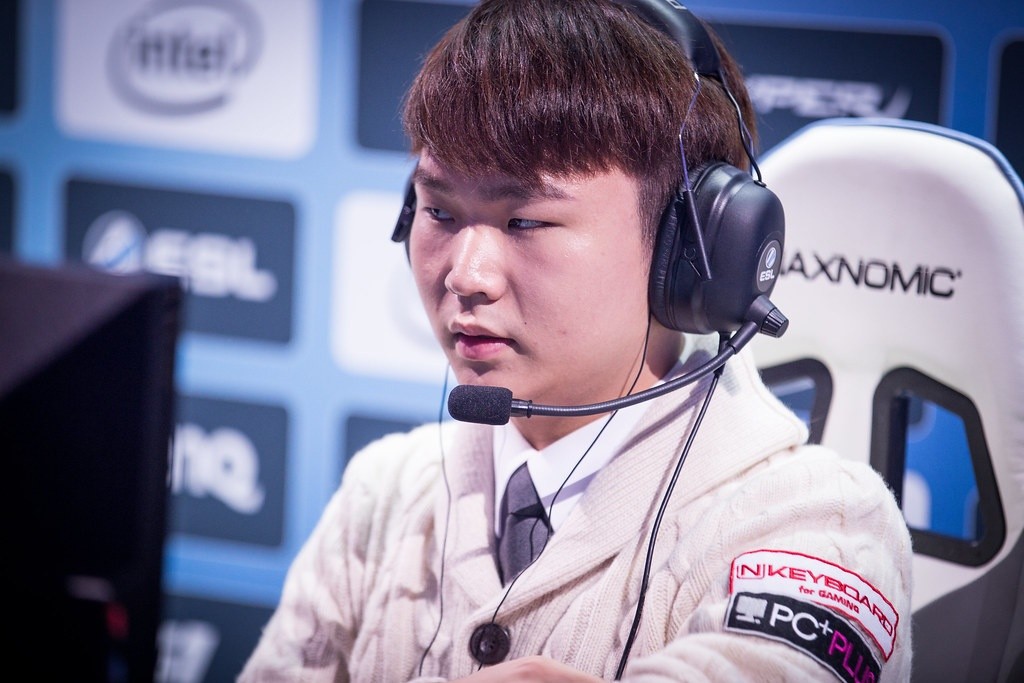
[238,0,916,683]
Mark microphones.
[449,320,758,426]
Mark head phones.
[387,0,788,340]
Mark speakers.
[0,259,184,682]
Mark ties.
[497,465,550,586]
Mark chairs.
[687,117,1024,682]
